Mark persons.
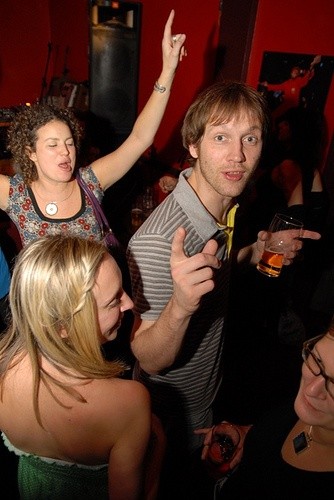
[193,323,334,500]
[0,10,187,249]
[239,86,327,311]
[128,82,321,500]
[0,234,167,500]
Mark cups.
[142,186,154,209]
[205,423,241,482]
[255,214,304,278]
[131,208,142,226]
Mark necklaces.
[292,426,334,456]
[34,180,75,217]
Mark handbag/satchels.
[76,173,127,268]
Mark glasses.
[301,333,334,401]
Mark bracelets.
[154,80,174,93]
